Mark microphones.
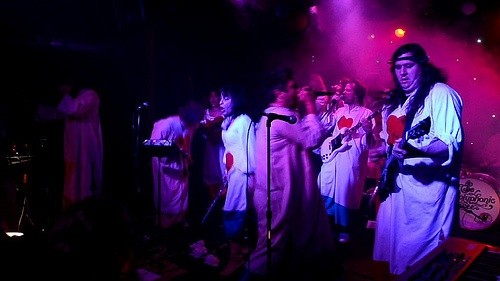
[337,95,345,102]
[264,113,297,124]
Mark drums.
[456,172,500,231]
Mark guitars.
[320,104,391,163]
[358,116,431,231]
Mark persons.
[54,75,103,230]
[150,68,375,281]
[373,44,464,281]
